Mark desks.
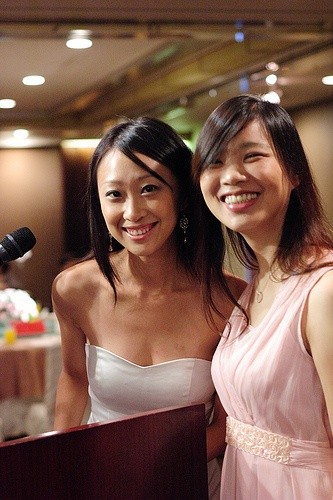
[0,313,61,443]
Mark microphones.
[0,227,36,268]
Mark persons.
[192,95,333,500]
[51,115,251,500]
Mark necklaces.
[254,264,276,303]
